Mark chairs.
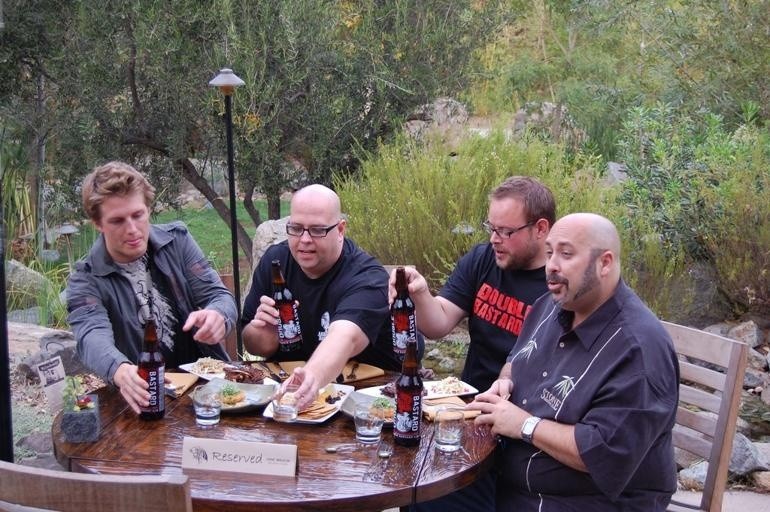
[0,457,195,512]
[659,319,751,512]
[213,273,239,361]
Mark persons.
[387,174,557,396]
[240,184,425,412]
[400,211,682,512]
[65,159,239,415]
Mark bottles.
[389,265,416,365]
[270,258,302,350]
[393,338,421,447]
[137,317,166,422]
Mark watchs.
[520,416,542,443]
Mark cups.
[432,408,463,452]
[192,385,222,424]
[353,402,384,445]
[271,383,299,425]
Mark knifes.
[272,360,291,380]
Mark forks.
[257,361,282,383]
[345,363,361,380]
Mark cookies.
[222,391,246,404]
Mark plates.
[188,376,277,414]
[179,358,282,406]
[409,379,479,401]
[339,383,397,424]
[263,383,356,424]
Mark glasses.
[285,219,340,238]
[482,218,536,240]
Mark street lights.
[209,66,249,363]
[56,219,81,275]
